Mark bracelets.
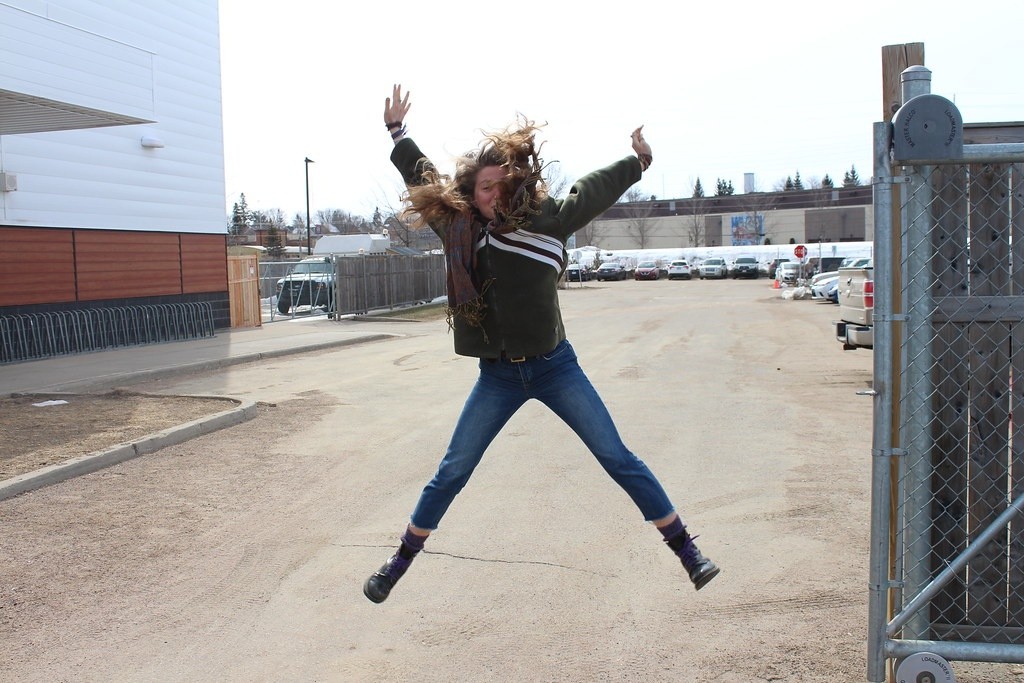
[384,122,407,139]
[638,153,652,171]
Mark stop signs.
[794,245,807,260]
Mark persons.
[363,83,719,605]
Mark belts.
[485,351,529,363]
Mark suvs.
[732,257,759,279]
[275,257,340,314]
[700,256,727,280]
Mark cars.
[767,256,873,305]
[667,260,693,280]
[596,263,627,281]
[634,260,660,280]
[564,263,592,282]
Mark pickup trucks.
[831,266,873,352]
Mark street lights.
[305,156,314,256]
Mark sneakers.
[666,529,720,590]
[363,545,419,603]
